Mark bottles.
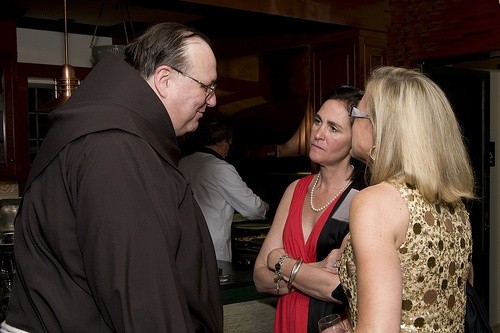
[0,198,23,232]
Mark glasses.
[226,138,232,149]
[348,105,373,121]
[172,65,217,100]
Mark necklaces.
[310,165,351,212]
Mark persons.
[322,66,483,333]
[178,122,269,286]
[253,84,372,333]
[4,23,224,333]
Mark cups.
[318,314,348,333]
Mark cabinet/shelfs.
[246,37,391,159]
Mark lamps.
[36,0,81,115]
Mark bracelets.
[275,254,290,276]
[275,277,282,293]
[287,260,302,286]
